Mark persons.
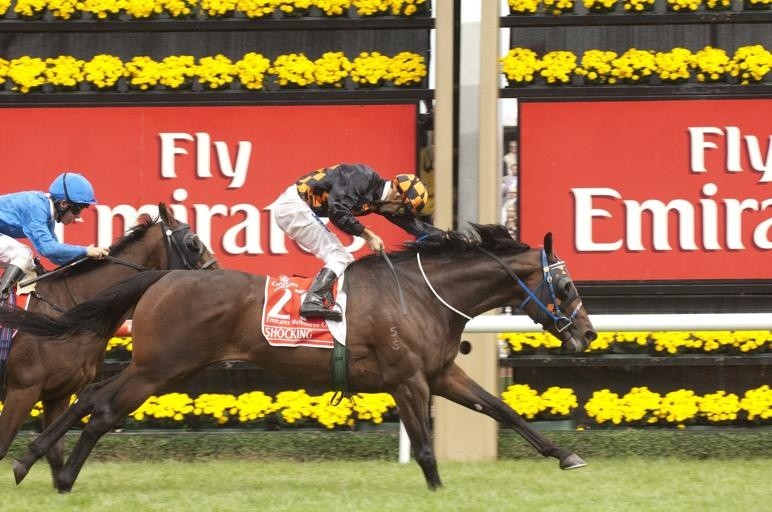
[273,161,427,320]
[0,170,111,306]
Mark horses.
[0,220,598,495]
[0,200,222,493]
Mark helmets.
[47,172,97,206]
[391,172,429,218]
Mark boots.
[0,262,25,302]
[299,268,342,322]
